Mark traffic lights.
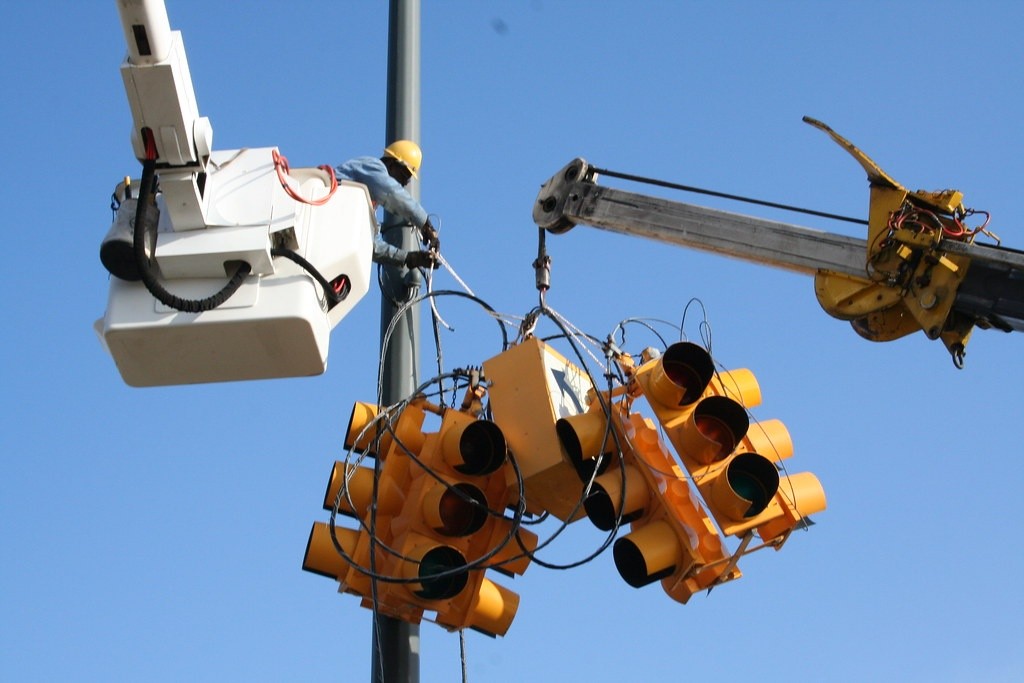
[556,341,828,605]
[301,384,546,639]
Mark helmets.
[384,139,422,180]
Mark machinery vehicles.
[533,115,1024,370]
[93,1,379,390]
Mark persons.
[333,141,440,270]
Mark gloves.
[406,251,439,269]
[421,220,440,253]
